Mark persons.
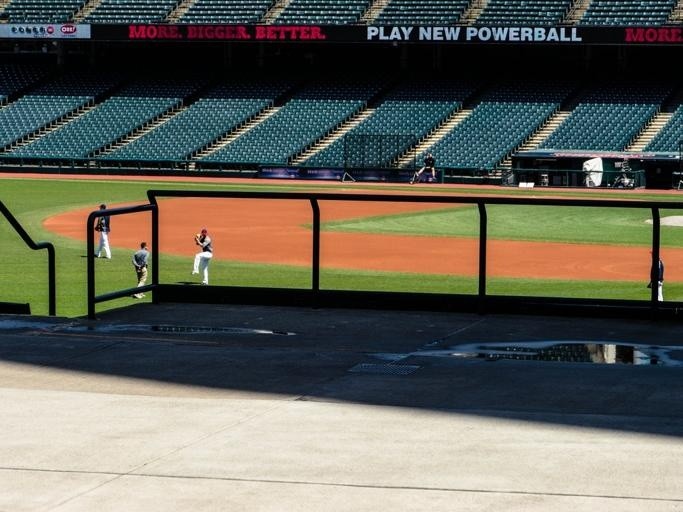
[95,204,112,259]
[131,241,149,299]
[646,251,664,302]
[415,153,437,179]
[191,228,213,286]
[616,171,635,188]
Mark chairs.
[373,0,473,26]
[0,1,96,23]
[644,103,683,154]
[575,0,679,26]
[473,0,575,25]
[81,0,186,25]
[197,96,367,172]
[300,99,462,170]
[537,101,659,152]
[98,98,274,172]
[403,99,559,169]
[0,95,94,151]
[176,0,278,24]
[270,0,375,24]
[6,97,185,165]
